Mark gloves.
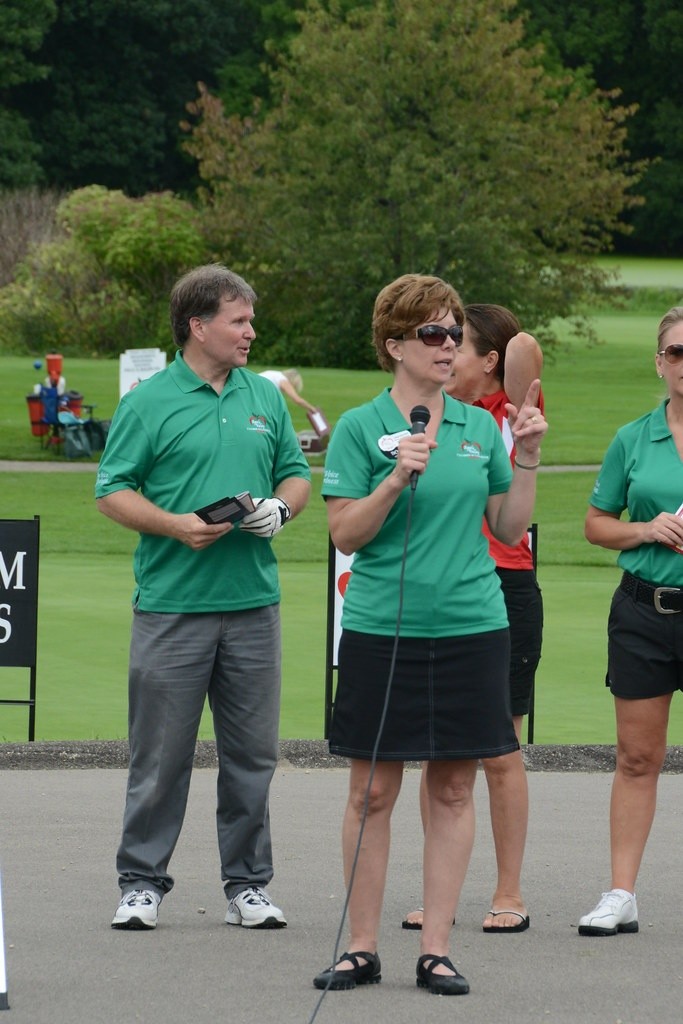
[238,492,291,538]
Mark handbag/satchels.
[86,419,112,452]
[57,412,94,459]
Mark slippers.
[402,907,456,930]
[483,910,529,933]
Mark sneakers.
[579,893,640,936]
[109,888,161,929]
[224,888,288,929]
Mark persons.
[95,267,313,929]
[312,274,548,995]
[254,367,316,413]
[578,307,683,936]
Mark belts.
[620,571,683,616]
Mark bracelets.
[514,457,541,470]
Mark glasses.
[395,324,463,347]
[659,343,683,366]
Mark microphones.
[410,406,431,490]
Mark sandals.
[416,952,470,995]
[314,950,383,991]
